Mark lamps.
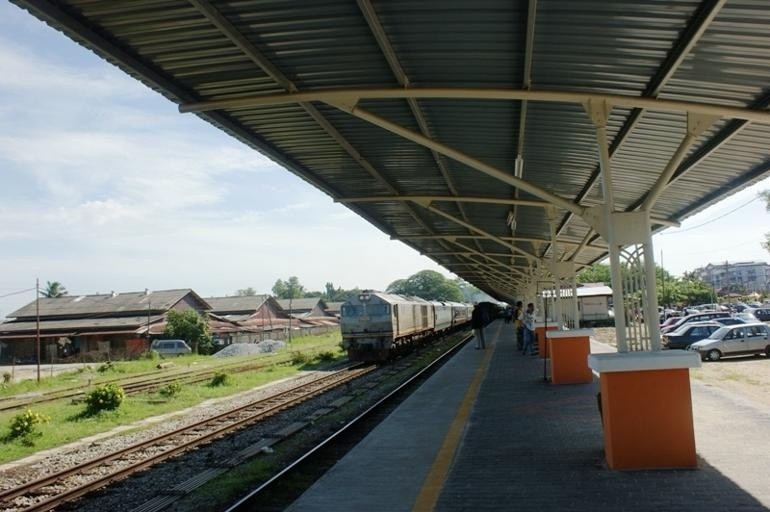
[515,152,523,179]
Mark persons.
[469,300,486,350]
[494,301,536,356]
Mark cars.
[689,323,770,362]
[635,292,770,352]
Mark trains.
[340,289,502,365]
[198,320,339,357]
[475,301,505,324]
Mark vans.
[150,339,192,359]
